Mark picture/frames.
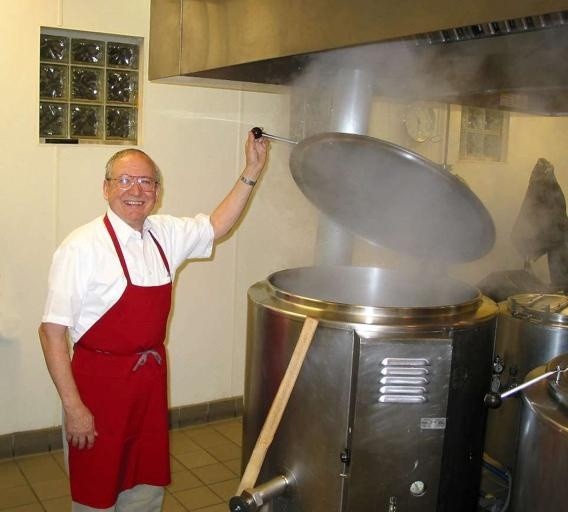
[445,103,510,167]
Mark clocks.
[401,100,437,143]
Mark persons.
[38,132,271,512]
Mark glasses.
[107,178,160,190]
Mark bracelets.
[238,175,258,187]
